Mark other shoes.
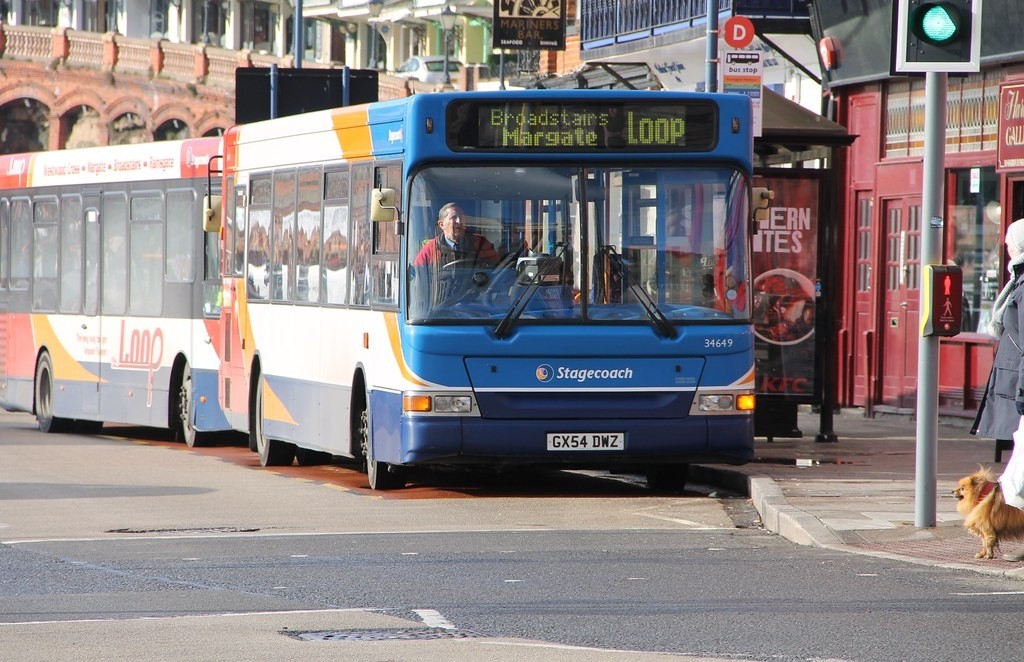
[1003,541,1024,562]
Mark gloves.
[1015,401,1024,416]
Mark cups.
[753,268,816,345]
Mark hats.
[1004,218,1024,257]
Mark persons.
[412,201,500,269]
[970,218,1024,562]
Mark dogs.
[952,463,1023,559]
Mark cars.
[390,53,465,87]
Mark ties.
[455,243,462,259]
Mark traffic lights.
[893,0,981,74]
[925,263,960,338]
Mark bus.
[214,90,776,491]
[1,135,247,449]
